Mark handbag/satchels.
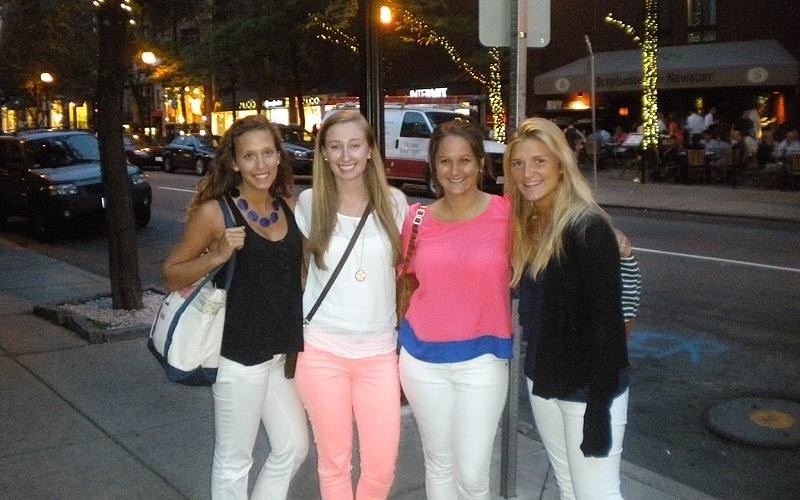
[282,350,298,379]
[148,274,227,385]
[395,272,420,355]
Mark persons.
[163,114,311,500]
[393,118,514,500]
[564,103,799,190]
[291,108,414,500]
[500,114,630,500]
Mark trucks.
[320,97,513,201]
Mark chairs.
[575,129,800,184]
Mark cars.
[99,118,325,177]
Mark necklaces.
[232,185,282,228]
[333,216,370,283]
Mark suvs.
[1,132,157,239]
